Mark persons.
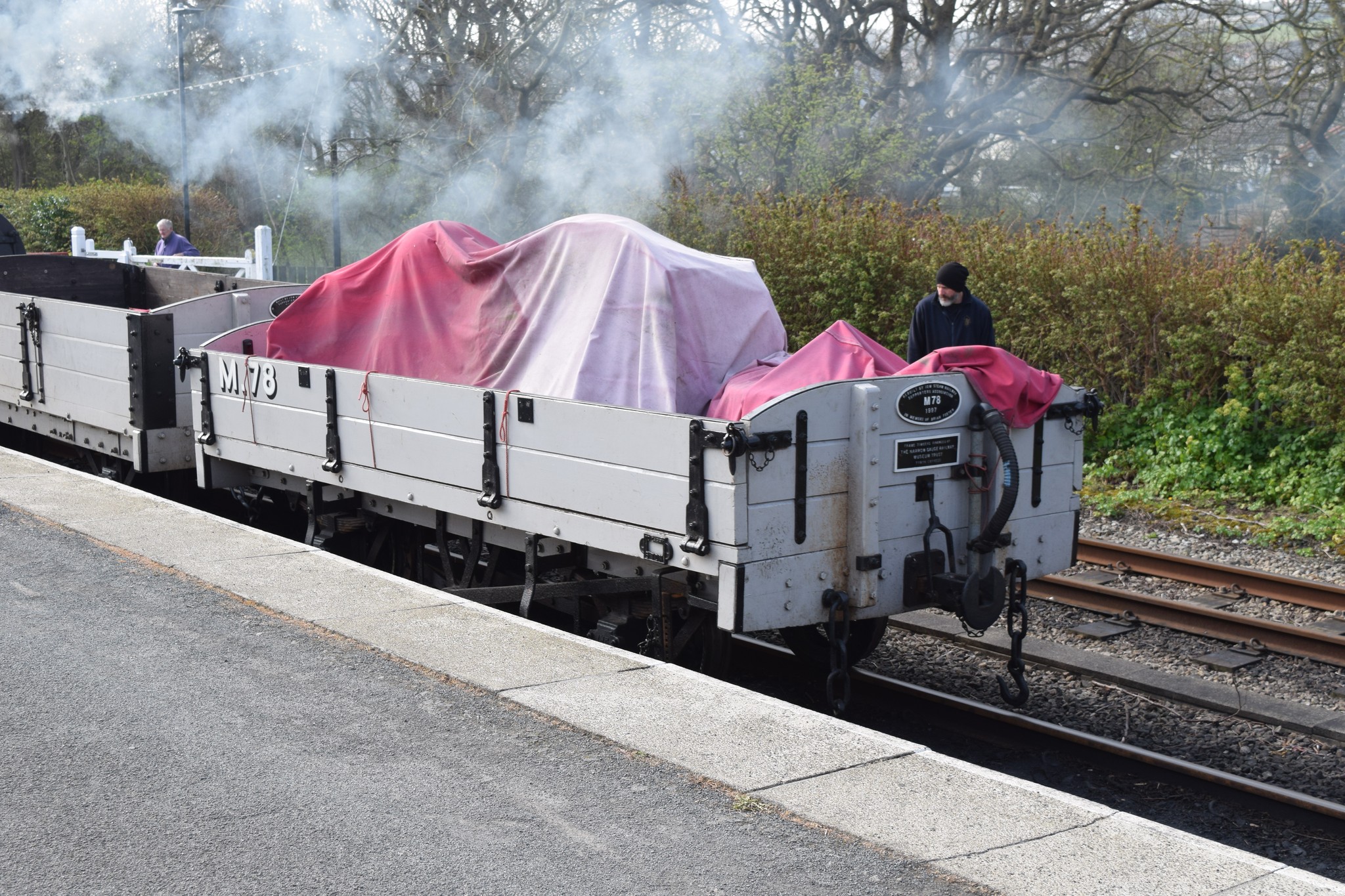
[152,218,202,271]
[907,262,996,365]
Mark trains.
[0,223,1102,715]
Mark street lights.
[172,1,205,242]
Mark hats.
[935,261,970,292]
